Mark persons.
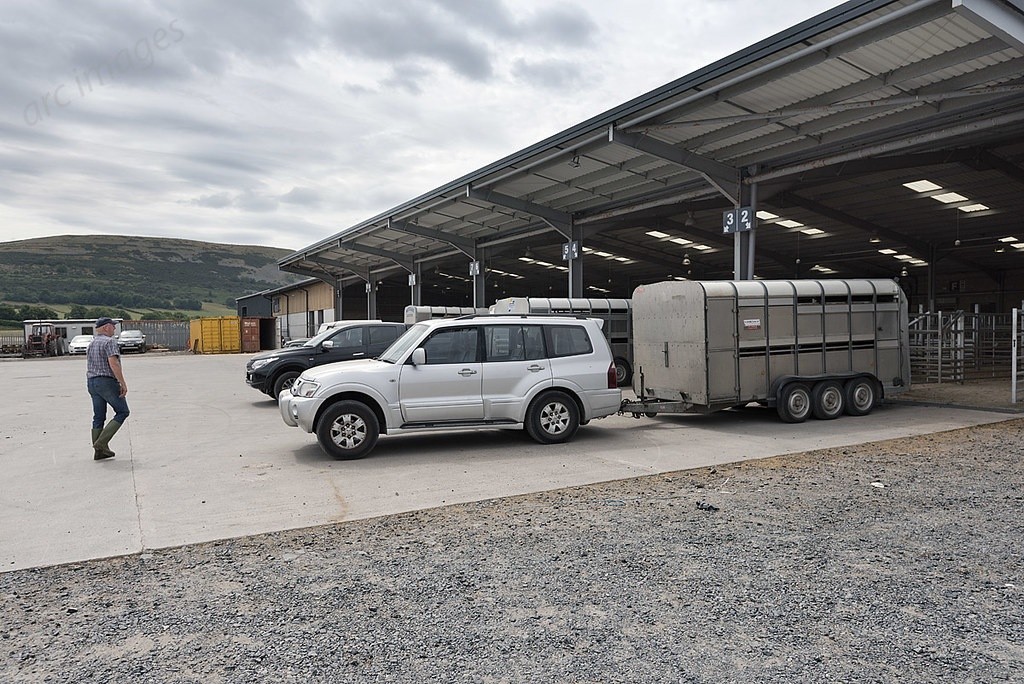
[86,317,129,460]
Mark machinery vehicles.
[21,323,65,359]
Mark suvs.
[245,322,478,402]
[281,319,383,349]
[277,314,622,460]
[115,329,147,354]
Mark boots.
[92,419,121,456]
[92,429,109,460]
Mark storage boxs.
[190,316,260,353]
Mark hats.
[95,318,118,328]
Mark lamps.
[681,254,691,266]
[568,156,581,169]
[901,263,908,276]
[525,217,534,257]
[869,233,881,242]
[685,202,697,226]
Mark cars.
[68,335,96,355]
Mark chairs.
[451,332,476,361]
[510,330,528,360]
